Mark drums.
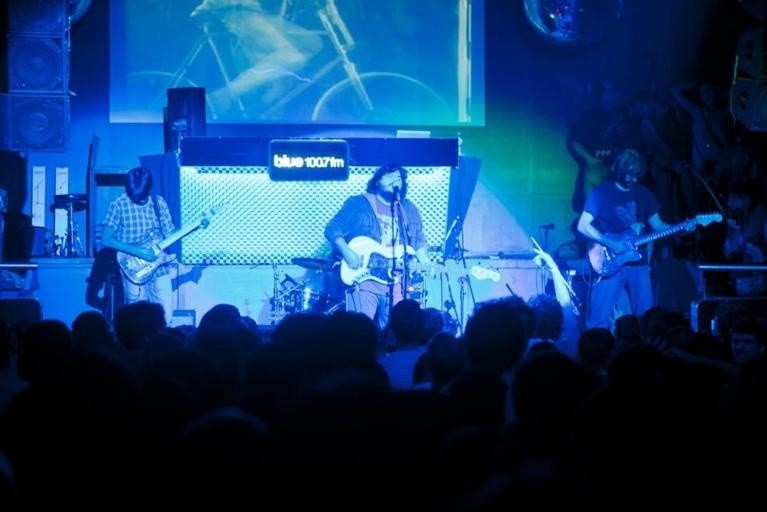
[290,288,346,316]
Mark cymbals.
[291,258,335,272]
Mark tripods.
[453,229,476,329]
[440,270,464,335]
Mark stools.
[54,194,89,257]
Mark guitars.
[587,211,723,276]
[340,235,500,286]
[116,208,218,284]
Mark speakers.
[0,264,43,329]
[0,0,70,154]
[690,298,767,346]
[167,87,206,150]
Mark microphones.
[392,181,401,201]
[444,214,460,240]
[539,223,554,230]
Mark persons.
[576,146,697,330]
[324,163,442,321]
[669,78,737,167]
[195,0,330,123]
[101,166,210,329]
[567,74,644,252]
